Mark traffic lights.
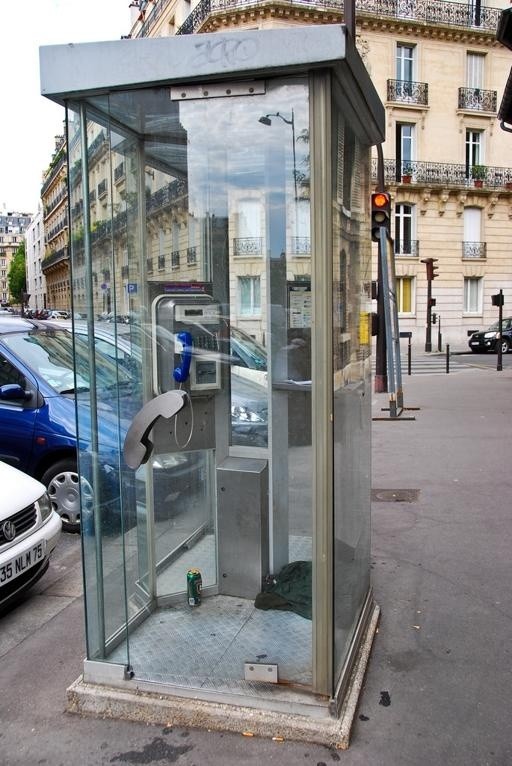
[434,313,439,323]
[490,294,498,307]
[370,191,391,242]
[432,258,439,280]
[24,293,31,302]
[361,282,374,299]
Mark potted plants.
[470,165,487,188]
[401,167,412,183]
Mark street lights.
[257,108,301,252]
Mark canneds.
[187,569,202,608]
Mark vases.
[506,183,512,190]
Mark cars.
[466,315,512,354]
[0,457,67,621]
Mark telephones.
[151,294,222,403]
[124,390,187,470]
